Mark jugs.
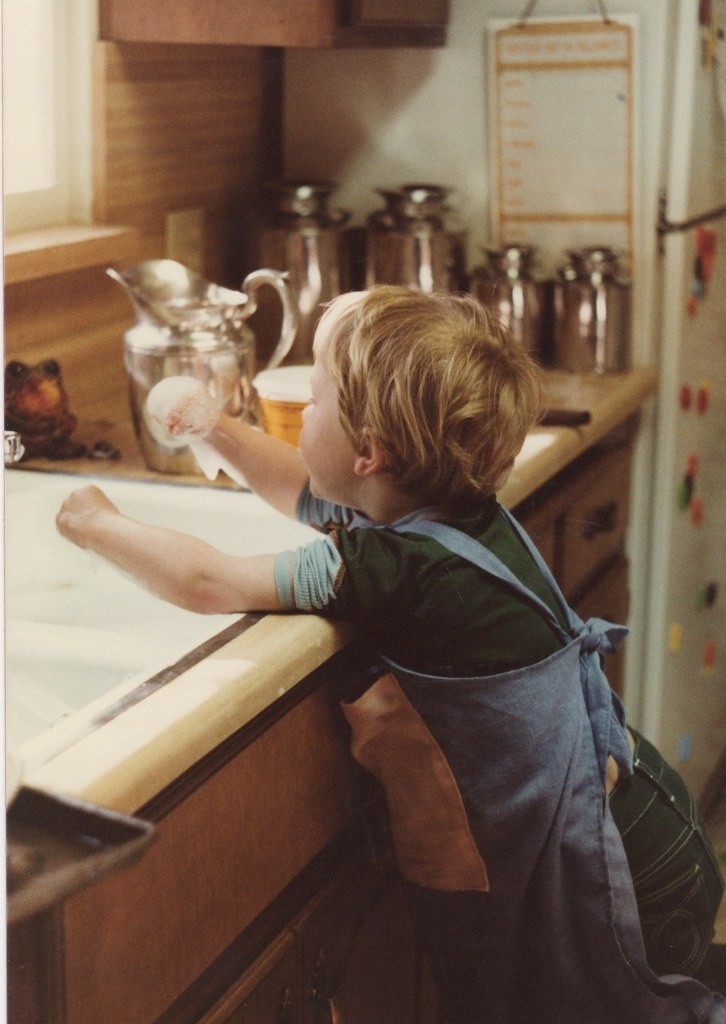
[251,174,625,376]
[104,255,303,474]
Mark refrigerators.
[282,0,726,826]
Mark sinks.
[4,467,316,786]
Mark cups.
[251,364,315,450]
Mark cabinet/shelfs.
[98,0,452,53]
[7,412,651,1024]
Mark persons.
[56,283,726,1024]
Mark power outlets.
[168,206,205,275]
